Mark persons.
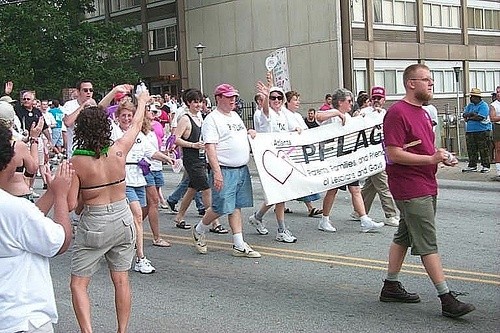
[66,90,150,333]
[490,85,500,177]
[0,64,475,333]
[461,88,493,173]
[191,84,262,258]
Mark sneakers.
[249,212,269,235]
[318,218,337,232]
[359,217,385,233]
[135,257,155,273]
[379,278,420,304]
[437,289,477,317]
[275,228,298,243]
[191,223,208,254]
[231,243,262,258]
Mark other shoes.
[151,199,228,247]
[350,212,361,220]
[384,217,399,227]
[462,166,500,180]
[29,188,41,199]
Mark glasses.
[269,96,283,101]
[371,97,384,101]
[83,88,94,92]
[23,98,31,100]
[407,77,434,82]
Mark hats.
[0,96,17,121]
[267,87,286,109]
[371,86,385,97]
[215,84,240,97]
[468,86,486,97]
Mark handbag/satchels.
[128,158,151,176]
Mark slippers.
[308,208,323,216]
[274,208,292,213]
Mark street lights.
[193,43,207,96]
[451,63,464,157]
